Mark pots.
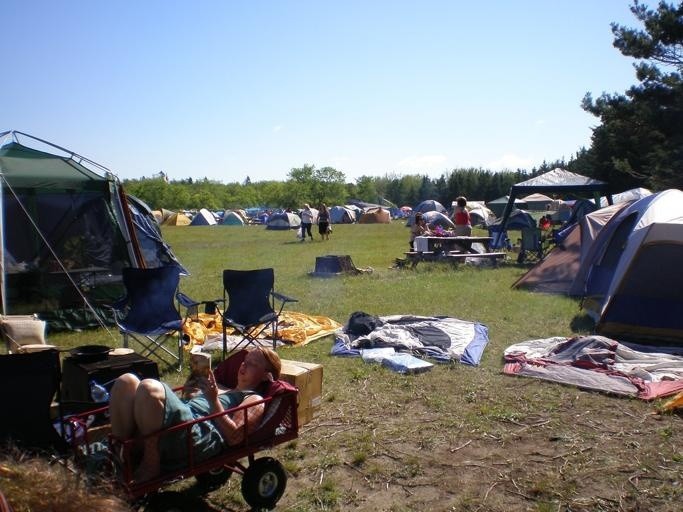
[70,345,115,363]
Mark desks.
[48,266,112,333]
[410,236,499,269]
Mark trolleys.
[68,350,299,509]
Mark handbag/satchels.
[345,311,384,335]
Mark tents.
[152,199,495,229]
[489,166,683,347]
[0,130,191,333]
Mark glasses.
[419,218,424,221]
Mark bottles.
[88,379,109,402]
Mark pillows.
[1,320,46,349]
[213,349,298,451]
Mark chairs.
[488,226,511,251]
[0,313,57,354]
[111,265,299,372]
[517,227,550,263]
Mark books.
[189,344,212,389]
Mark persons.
[316,203,330,240]
[409,196,478,255]
[110,346,282,485]
[300,204,313,242]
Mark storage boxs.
[279,359,323,428]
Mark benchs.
[403,248,506,269]
